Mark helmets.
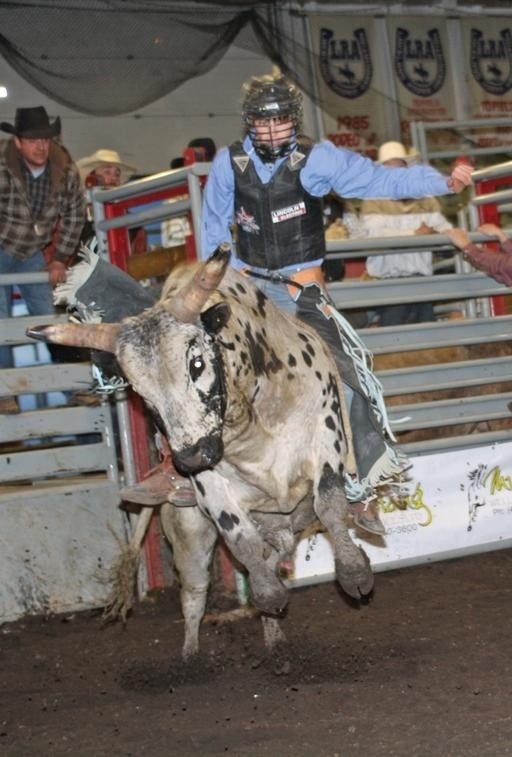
[243,76,304,164]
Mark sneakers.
[0,400,20,414]
[70,392,99,406]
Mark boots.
[350,499,384,535]
[119,436,197,507]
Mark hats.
[170,138,216,169]
[0,107,63,138]
[374,142,417,164]
[72,150,138,192]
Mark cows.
[24,239,376,679]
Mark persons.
[319,141,471,332]
[118,74,472,534]
[2,106,101,413]
[73,150,144,263]
[458,222,511,287]
[131,137,217,253]
